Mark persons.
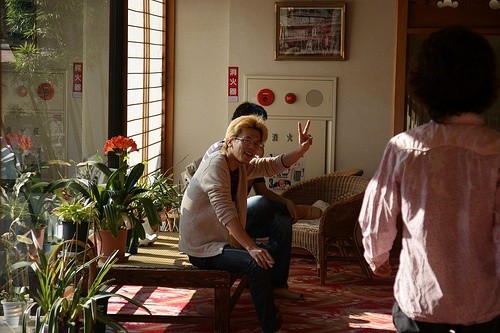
[358,27,500,333]
[198,102,305,300]
[178,115,313,333]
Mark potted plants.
[0,164,187,333]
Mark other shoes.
[294,205,322,220]
[273,288,305,300]
[275,312,284,321]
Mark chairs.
[277,168,373,283]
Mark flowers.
[103,135,139,188]
[6,134,32,168]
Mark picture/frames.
[274,2,347,61]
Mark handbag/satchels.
[306,200,330,225]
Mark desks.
[91,232,256,333]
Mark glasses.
[235,137,264,150]
